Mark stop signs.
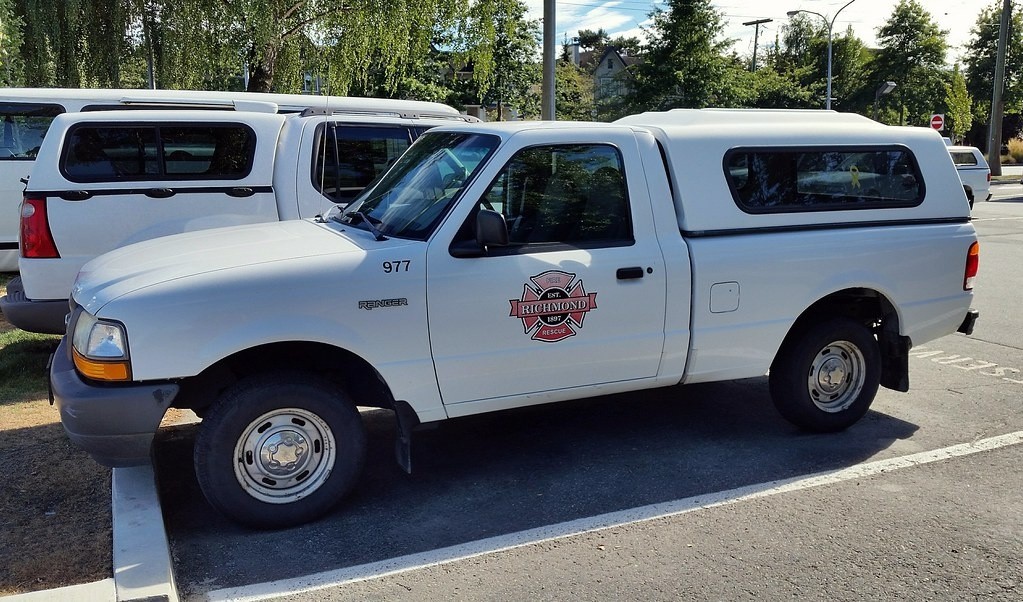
[930,113,944,132]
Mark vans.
[0,87,468,276]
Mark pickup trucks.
[796,146,991,214]
[45,105,981,530]
[0,97,480,340]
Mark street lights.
[874,82,896,121]
[787,10,831,110]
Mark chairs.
[167,149,205,180]
[509,166,632,243]
[73,139,127,179]
